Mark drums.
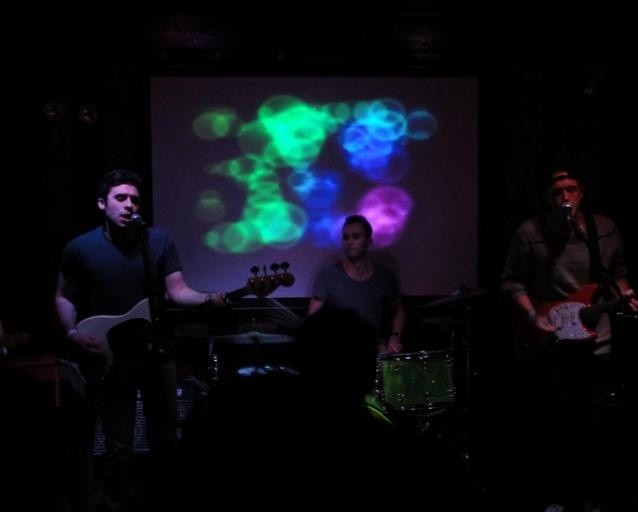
[374,346,457,410]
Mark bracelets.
[205,294,212,303]
[390,331,403,337]
[528,309,537,320]
[66,328,78,338]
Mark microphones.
[130,213,147,229]
[560,202,586,243]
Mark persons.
[307,214,407,354]
[55,169,234,351]
[500,168,638,370]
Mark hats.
[546,162,578,185]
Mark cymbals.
[213,320,293,344]
[237,363,297,377]
[424,290,478,310]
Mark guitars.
[538,280,638,349]
[72,261,294,376]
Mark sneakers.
[543,500,564,511]
[95,483,122,510]
[579,497,602,511]
